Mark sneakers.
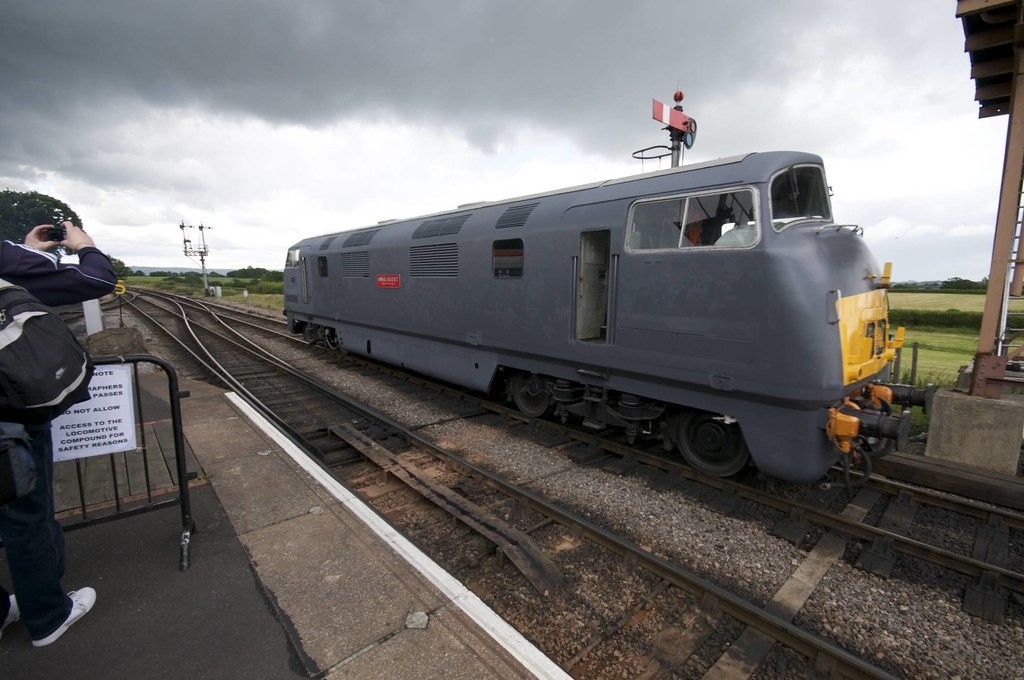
[2,594,20,627]
[31,587,97,648]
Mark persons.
[0,221,119,647]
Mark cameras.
[48,228,67,242]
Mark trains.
[281,150,938,483]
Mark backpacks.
[0,278,94,423]
